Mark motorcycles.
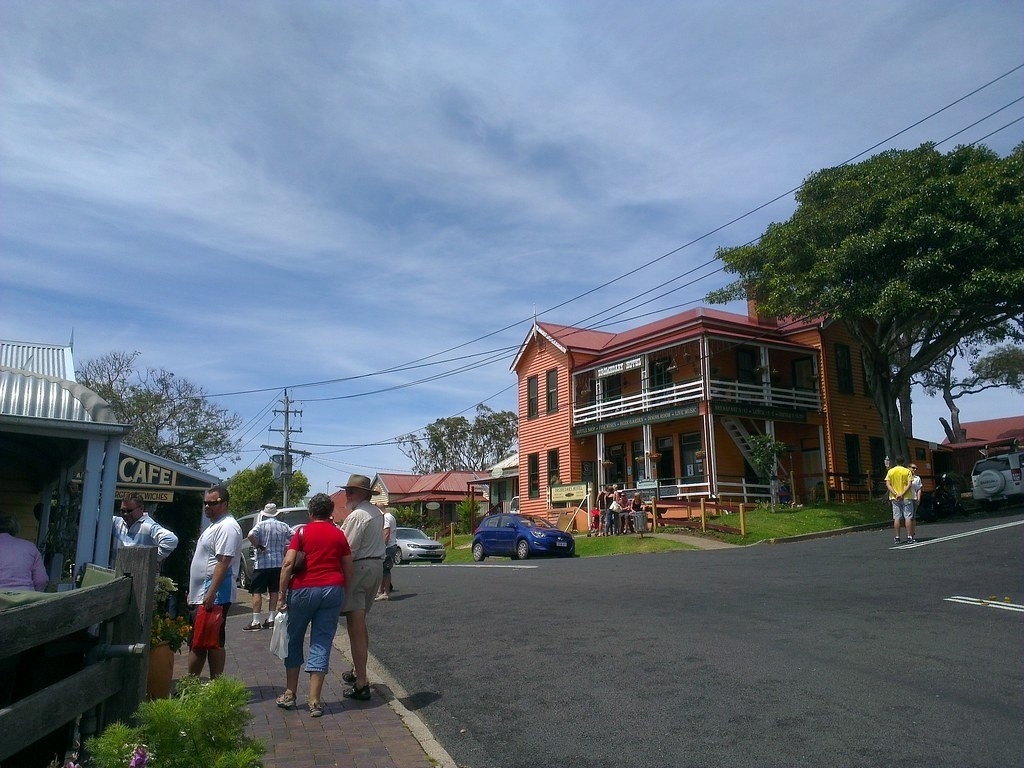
[917,473,970,523]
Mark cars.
[471,510,576,561]
[394,527,447,565]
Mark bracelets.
[278,591,286,593]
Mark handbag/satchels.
[609,500,623,513]
[191,604,223,652]
[383,557,393,571]
[269,611,288,661]
[284,526,306,575]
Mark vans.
[509,495,520,514]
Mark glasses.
[203,500,223,506]
[120,507,139,513]
[345,489,353,494]
[910,466,917,470]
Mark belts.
[352,556,381,562]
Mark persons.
[0,511,49,592]
[596,483,645,537]
[173,486,243,698]
[906,463,923,535]
[374,502,396,601]
[276,493,353,717]
[335,474,386,701]
[241,503,296,632]
[69,481,179,610]
[884,455,915,543]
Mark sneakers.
[307,702,322,717]
[907,535,917,543]
[894,537,901,544]
[341,669,357,684]
[261,619,274,629]
[374,595,391,600]
[242,622,263,631]
[342,683,371,700]
[275,691,296,707]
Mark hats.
[260,503,280,517]
[340,474,380,495]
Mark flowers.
[150,577,193,650]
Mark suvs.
[237,507,311,589]
[970,450,1024,512]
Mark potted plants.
[696,450,706,459]
[603,460,614,468]
[635,456,645,463]
[651,453,662,461]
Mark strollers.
[587,507,605,537]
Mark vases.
[148,640,174,699]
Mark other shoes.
[623,529,627,534]
[631,530,636,533]
[199,679,213,687]
[599,533,602,537]
